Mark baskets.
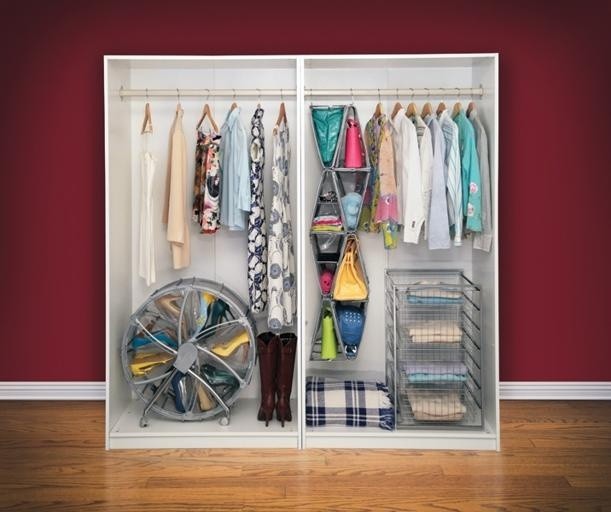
[387,271,481,431]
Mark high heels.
[128,294,249,412]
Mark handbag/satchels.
[344,120,362,168]
[332,238,368,301]
[342,192,362,229]
[321,305,338,360]
[315,108,342,164]
[338,305,364,358]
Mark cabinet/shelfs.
[104,54,500,453]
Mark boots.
[258,331,276,427]
[277,332,296,428]
